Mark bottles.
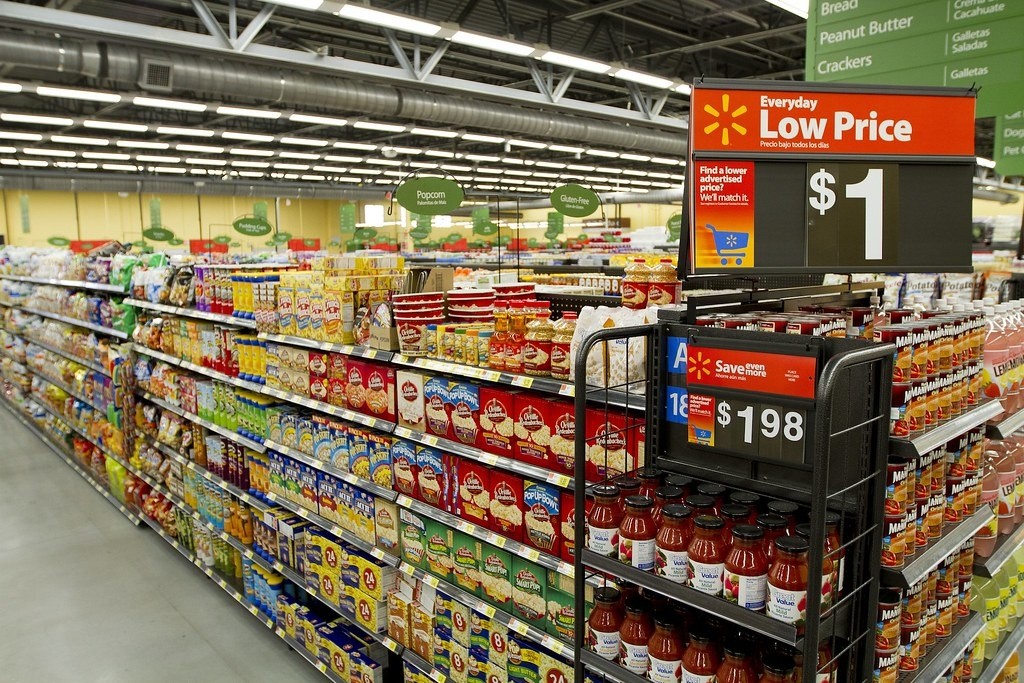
[427,300,576,380]
[587,461,844,683]
[870,294,1024,683]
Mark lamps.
[0,0,692,195]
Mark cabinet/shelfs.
[684,282,1006,683]
[0,273,141,527]
[120,294,645,683]
[575,320,899,683]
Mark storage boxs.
[169,253,646,682]
[971,406,1024,683]
